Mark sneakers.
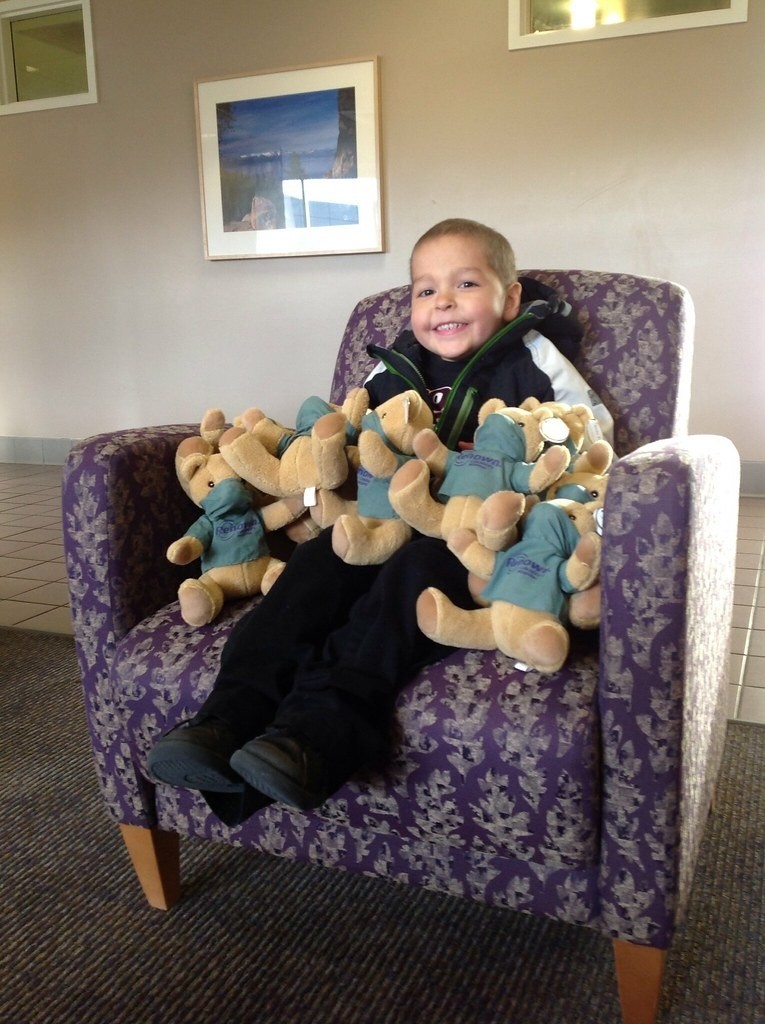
[146,719,244,795]
[230,726,329,811]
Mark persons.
[147,219,615,828]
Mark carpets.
[0,626,765,1024]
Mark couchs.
[59,272,740,1024]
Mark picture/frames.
[193,55,388,261]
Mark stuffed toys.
[166,386,614,676]
